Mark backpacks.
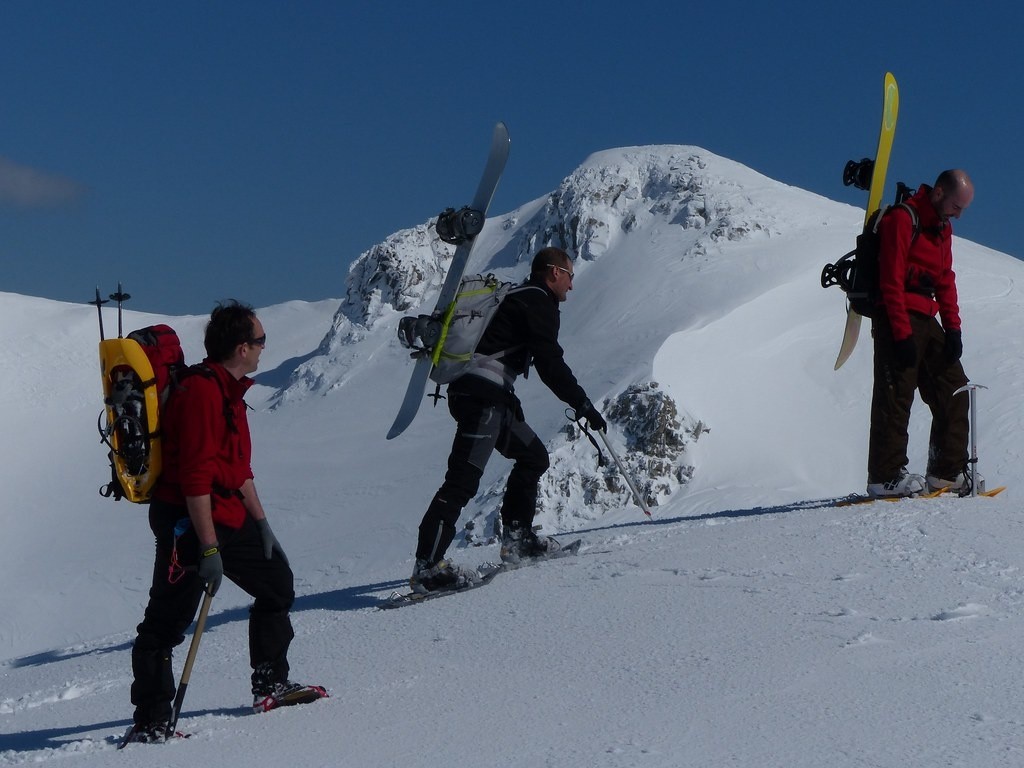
[849,202,921,319]
[430,272,551,385]
[114,321,232,476]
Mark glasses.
[544,261,574,281]
[241,334,267,346]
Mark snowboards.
[383,115,512,440]
[818,69,901,374]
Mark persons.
[866,168,986,496]
[409,248,607,588]
[125,297,329,742]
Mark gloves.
[942,326,962,361]
[897,334,916,368]
[574,402,607,435]
[198,544,224,598]
[256,514,292,564]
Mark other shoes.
[133,721,185,743]
[926,469,985,493]
[408,561,471,592]
[868,472,924,495]
[498,525,560,561]
[251,684,328,715]
